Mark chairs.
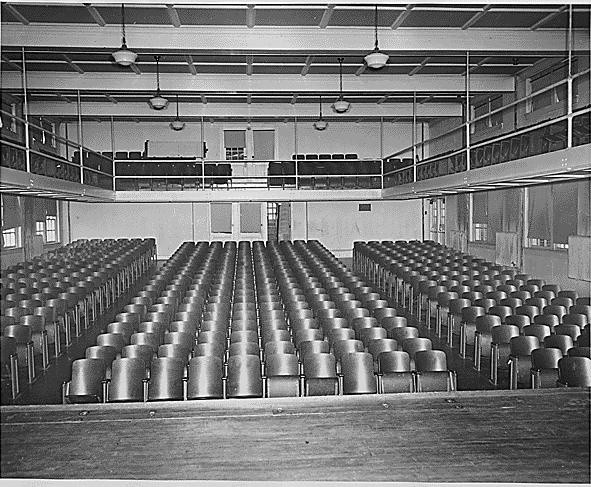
[267,104,590,190]
[0,128,233,190]
[0,238,158,400]
[61,239,457,405]
[354,240,591,388]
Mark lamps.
[314,95,328,130]
[149,55,168,109]
[364,5,389,69]
[171,94,185,130]
[333,57,350,112]
[111,4,138,66]
